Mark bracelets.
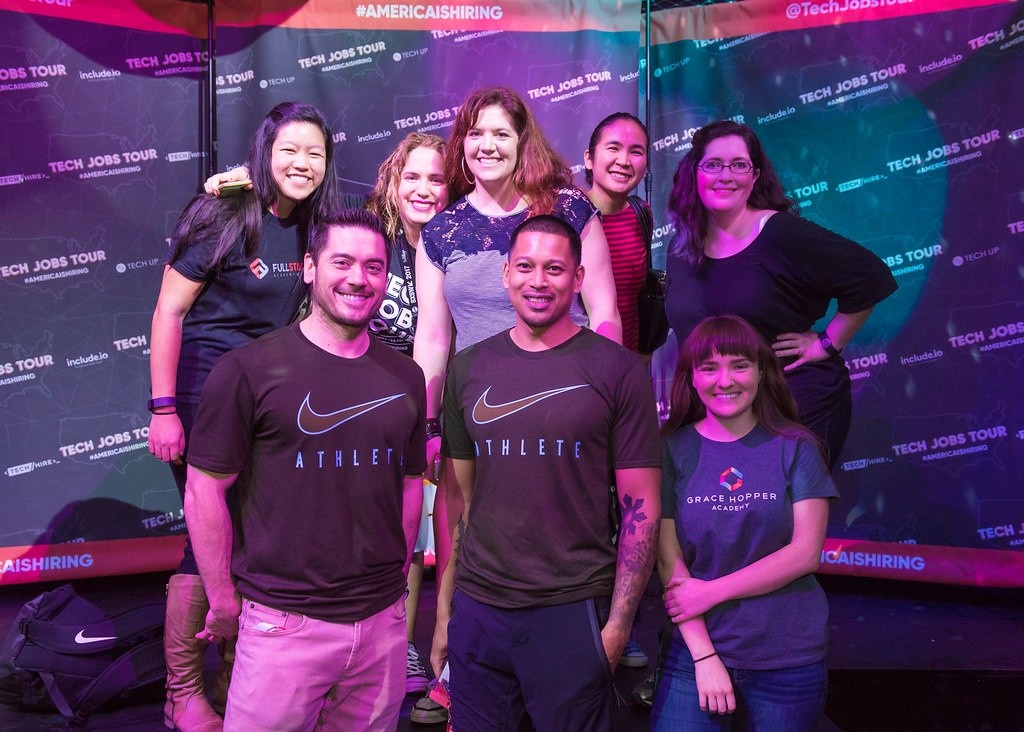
[424,418,443,433]
[426,431,443,442]
[147,396,178,409]
[818,329,842,357]
[149,408,176,416]
[693,652,718,664]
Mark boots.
[210,635,239,712]
[163,573,226,732]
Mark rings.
[793,347,802,358]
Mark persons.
[151,86,900,732]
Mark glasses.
[697,161,756,175]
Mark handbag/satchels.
[625,195,671,354]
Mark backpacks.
[0,573,221,732]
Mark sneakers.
[411,680,448,724]
[632,673,655,705]
[402,640,428,693]
[615,626,650,667]
